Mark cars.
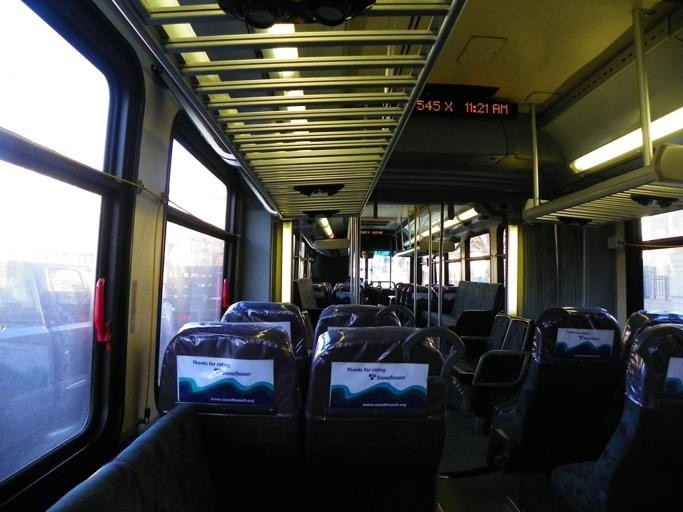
[171,294,221,329]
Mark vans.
[0,259,173,481]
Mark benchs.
[293,277,325,333]
[422,281,503,336]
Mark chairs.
[313,282,363,307]
[452,315,536,428]
[314,304,404,350]
[220,304,307,391]
[550,323,683,511]
[389,283,457,325]
[622,309,683,368]
[306,321,455,511]
[157,321,304,509]
[488,306,621,478]
